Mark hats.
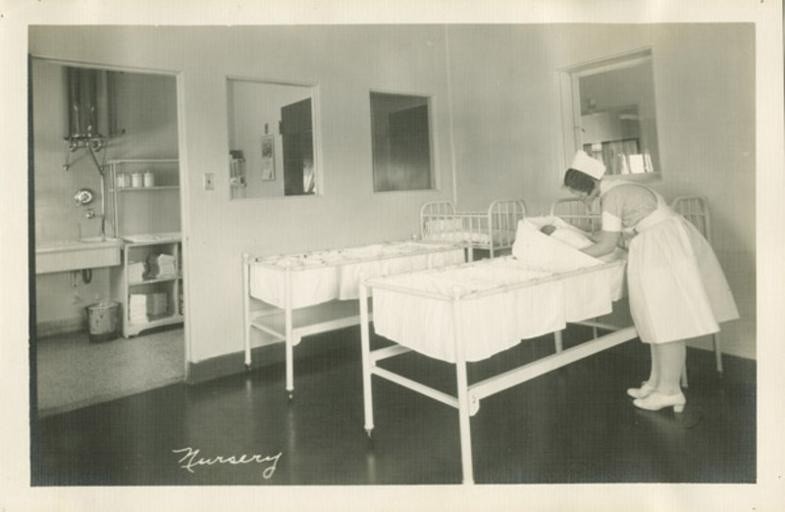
[565,150,607,180]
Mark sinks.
[35,237,121,274]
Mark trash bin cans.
[85,300,120,342]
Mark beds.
[243,192,724,485]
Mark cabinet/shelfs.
[101,157,183,339]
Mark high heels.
[632,392,686,414]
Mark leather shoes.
[626,380,657,398]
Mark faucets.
[85,208,103,219]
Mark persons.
[540,223,616,261]
[561,152,745,412]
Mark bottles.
[117,169,154,189]
